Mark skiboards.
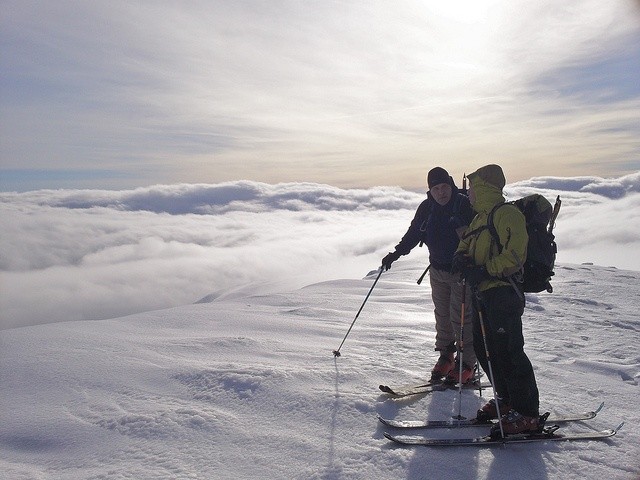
[376,402,626,446]
[379,368,492,396]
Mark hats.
[428,167,453,189]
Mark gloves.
[381,252,398,272]
[451,251,470,276]
[458,265,492,283]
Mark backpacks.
[486,194,558,294]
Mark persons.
[449,165,541,435]
[380,166,478,385]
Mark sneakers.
[445,363,475,384]
[478,396,509,419]
[431,353,455,378]
[489,409,539,434]
